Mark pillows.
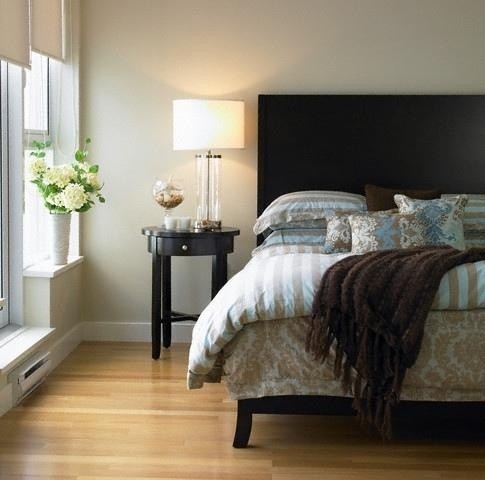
[249,182,485,259]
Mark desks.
[141,226,242,359]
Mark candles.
[165,217,190,229]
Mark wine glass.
[152,177,185,229]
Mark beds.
[224,91,485,450]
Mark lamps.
[172,98,245,230]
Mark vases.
[49,213,71,265]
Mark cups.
[179,216,191,231]
[163,216,177,230]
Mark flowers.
[26,135,105,215]
